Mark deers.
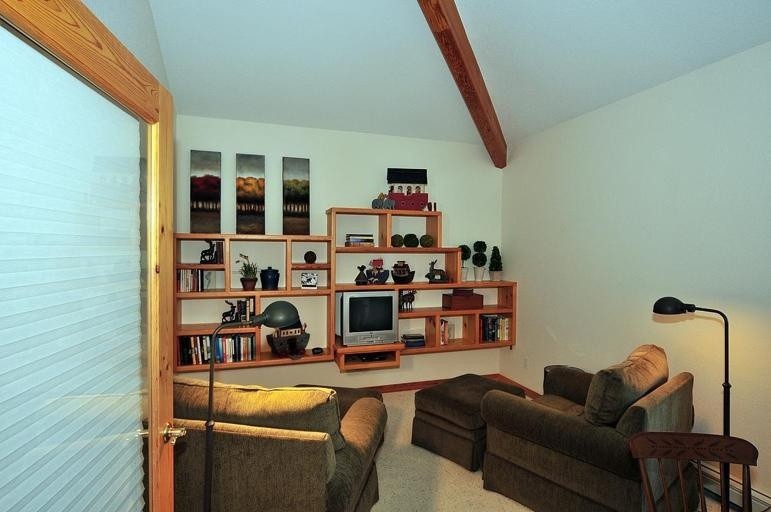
[222,300,236,323]
[201,239,215,261]
[429,259,445,280]
[401,289,418,310]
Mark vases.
[240,277,258,291]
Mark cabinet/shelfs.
[173,207,518,373]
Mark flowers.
[234,249,261,279]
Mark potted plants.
[456,244,471,281]
[487,245,505,282]
[472,240,488,282]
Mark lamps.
[654,296,732,512]
[199,298,303,511]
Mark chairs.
[174,374,387,511]
[627,429,761,512]
[478,342,696,509]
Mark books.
[177,333,256,366]
[401,338,427,348]
[344,232,376,248]
[445,318,449,345]
[479,314,513,343]
[403,334,425,339]
[440,319,445,346]
[176,268,217,292]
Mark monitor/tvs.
[340,291,399,347]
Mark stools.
[408,374,528,474]
[293,383,387,450]
